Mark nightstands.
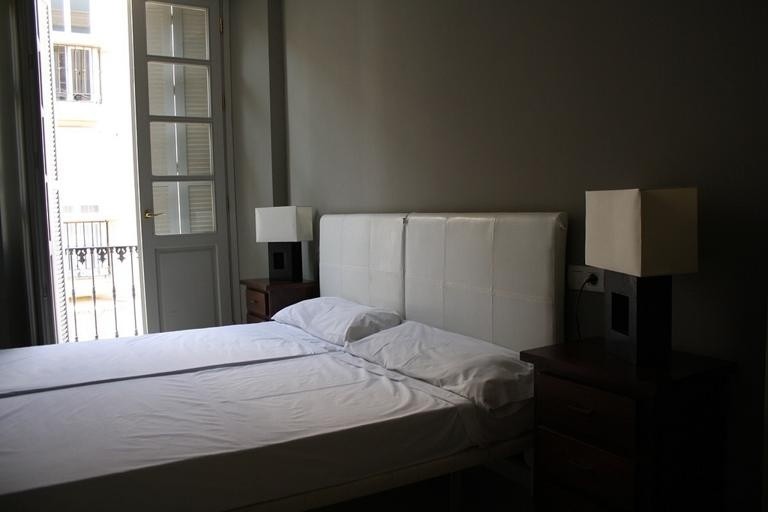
[521,342,736,512]
[240,279,313,322]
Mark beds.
[0,212,569,512]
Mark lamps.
[255,206,313,285]
[587,189,698,367]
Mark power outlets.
[568,263,605,293]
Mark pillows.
[348,322,534,409]
[271,297,404,346]
